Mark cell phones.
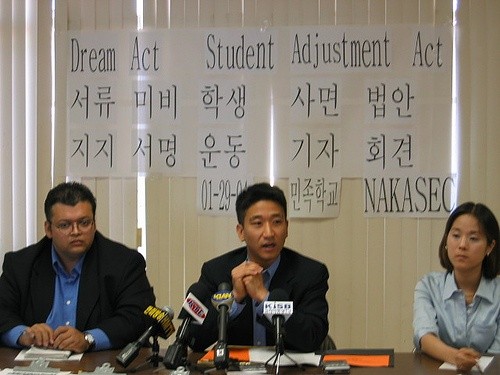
[320,360,351,375]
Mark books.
[321,348,395,368]
[24,346,73,359]
[196,345,250,365]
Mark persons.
[188,182,330,353]
[412,201,500,371]
[0,182,156,353]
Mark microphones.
[262,288,293,357]
[116,305,176,368]
[211,282,235,371]
[163,282,209,370]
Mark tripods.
[129,334,165,374]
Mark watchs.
[84,332,95,352]
